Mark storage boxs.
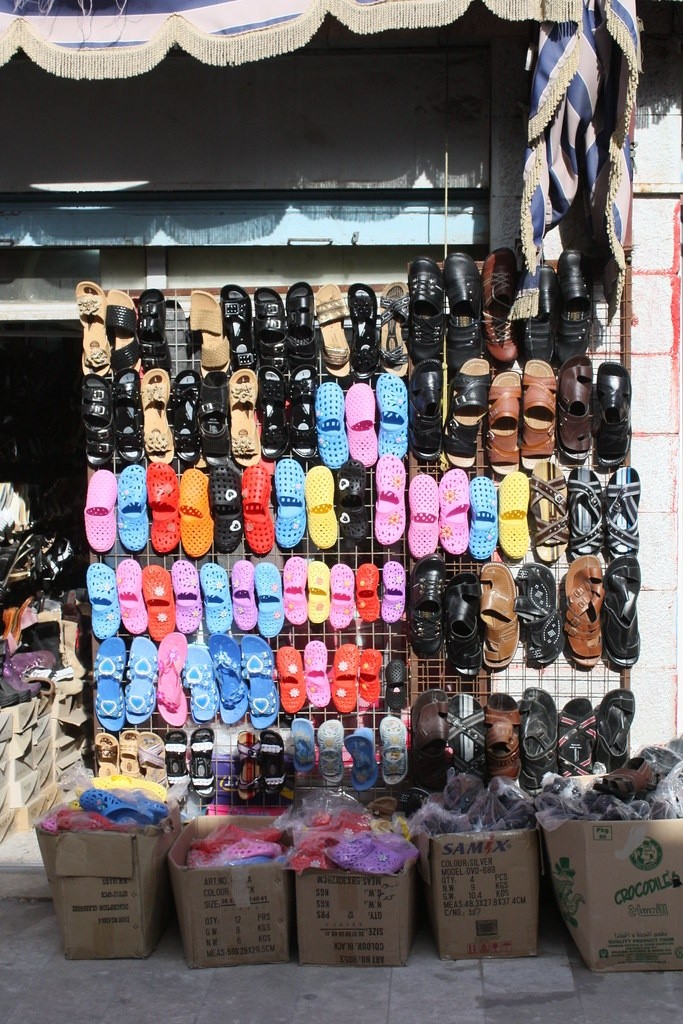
[32,804,683,973]
[0,603,93,845]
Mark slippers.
[0,239,683,875]
